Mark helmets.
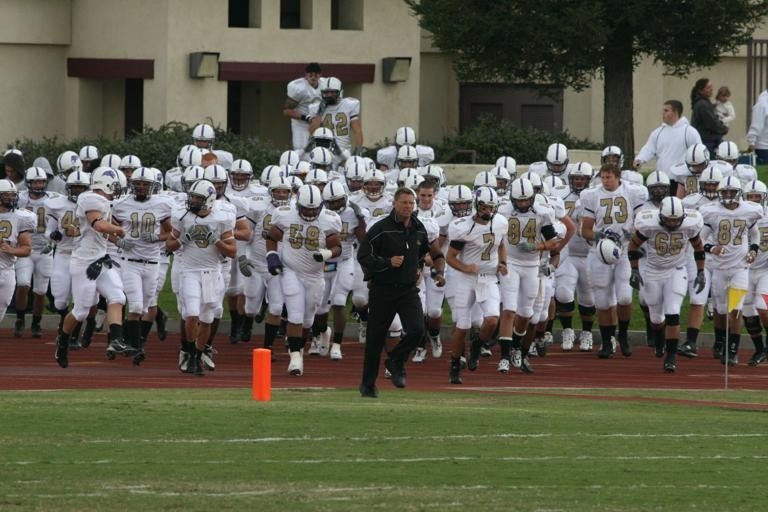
[191,124,216,140]
[313,127,333,140]
[0,141,767,221]
[395,127,416,145]
[596,238,621,265]
[320,77,342,92]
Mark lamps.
[381,56,413,85]
[188,49,221,80]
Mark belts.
[121,256,158,264]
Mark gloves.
[629,270,644,290]
[313,247,332,263]
[103,254,120,268]
[317,100,328,115]
[52,230,62,242]
[116,238,135,251]
[142,232,159,243]
[265,251,285,276]
[40,242,52,254]
[237,255,255,276]
[541,262,554,277]
[179,224,203,245]
[200,227,218,244]
[87,260,102,280]
[593,228,606,240]
[692,269,706,294]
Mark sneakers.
[14,313,25,337]
[58,309,105,350]
[429,333,443,358]
[308,326,343,360]
[664,359,676,373]
[706,297,715,319]
[676,340,699,358]
[385,355,406,387]
[746,348,768,366]
[646,324,665,357]
[359,384,378,398]
[528,329,554,357]
[355,312,368,343]
[578,330,593,351]
[275,317,289,345]
[178,338,219,377]
[497,347,534,375]
[31,313,42,338]
[467,334,497,371]
[156,310,169,341]
[411,347,427,362]
[560,327,576,351]
[105,337,145,365]
[229,311,253,344]
[255,299,269,324]
[264,345,277,362]
[449,350,466,384]
[54,334,69,368]
[287,347,304,377]
[597,334,618,358]
[712,341,738,367]
[618,332,633,357]
